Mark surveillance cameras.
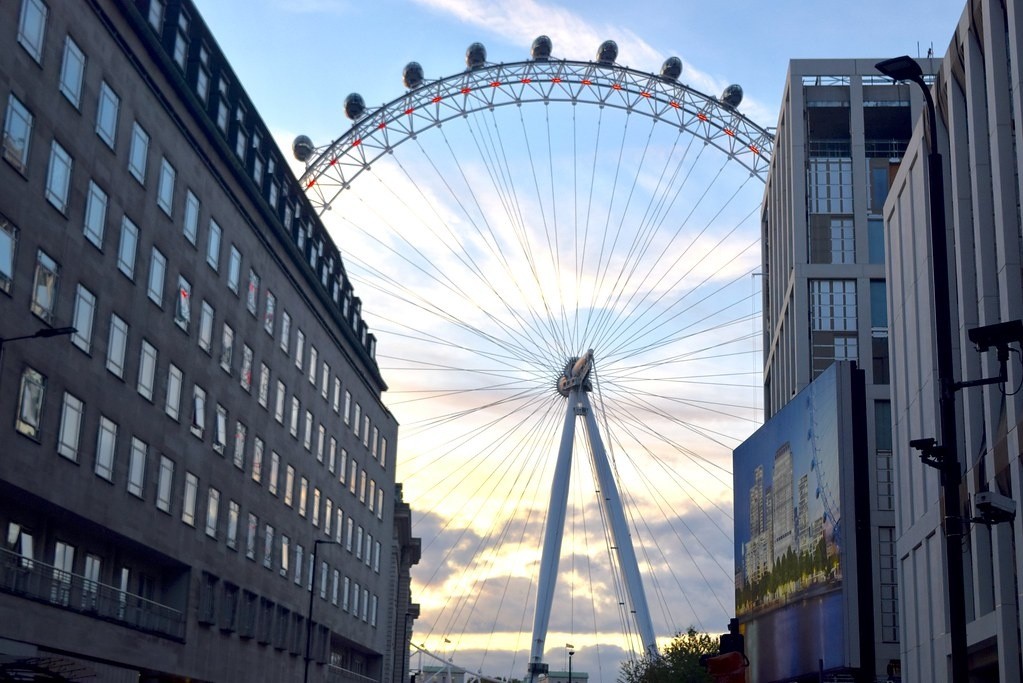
[974,491,1017,516]
[909,437,936,447]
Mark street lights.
[568,651,575,682]
[878,54,1023,683]
[305,540,342,683]
